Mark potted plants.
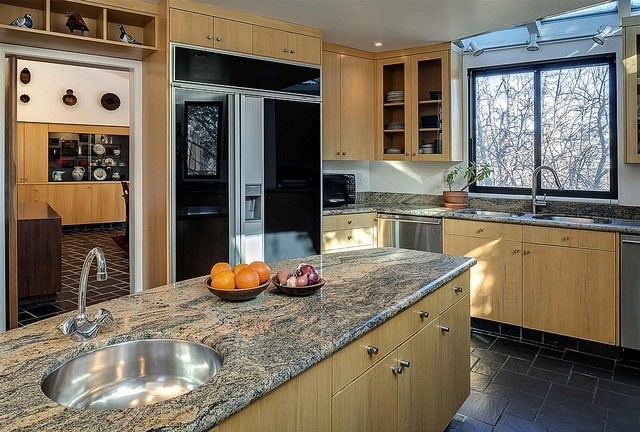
[443,160,494,209]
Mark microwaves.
[321,171,358,208]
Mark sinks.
[456,208,526,220]
[532,212,613,225]
[41,337,224,408]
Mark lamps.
[468,40,484,59]
[526,31,540,52]
[590,23,615,45]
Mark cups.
[114,150,121,155]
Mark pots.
[420,114,438,128]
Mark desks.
[15,202,62,305]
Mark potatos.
[296,272,308,286]
[276,269,296,286]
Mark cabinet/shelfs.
[17,122,130,226]
[322,41,375,160]
[142,1,322,67]
[374,42,463,163]
[440,268,471,430]
[442,219,619,349]
[332,290,441,432]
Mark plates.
[386,90,407,102]
[386,147,404,154]
[386,121,405,130]
[203,274,271,302]
[271,272,327,296]
[93,168,106,180]
[93,143,105,155]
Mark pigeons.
[9,13,33,28]
[117,24,145,45]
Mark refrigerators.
[169,42,323,287]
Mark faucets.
[61,245,114,337]
[530,164,564,213]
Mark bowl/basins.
[419,147,436,153]
[428,90,442,99]
[419,142,435,147]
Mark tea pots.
[52,171,65,181]
[72,166,85,181]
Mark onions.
[296,264,321,286]
